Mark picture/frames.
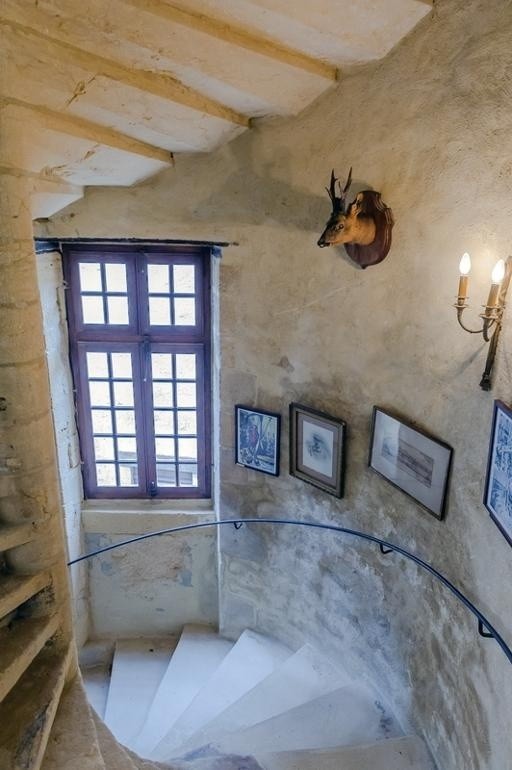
[233,402,347,500]
[482,399,512,547]
[367,404,453,522]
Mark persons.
[308,433,329,461]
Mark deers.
[316,166,376,249]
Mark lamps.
[453,253,512,391]
